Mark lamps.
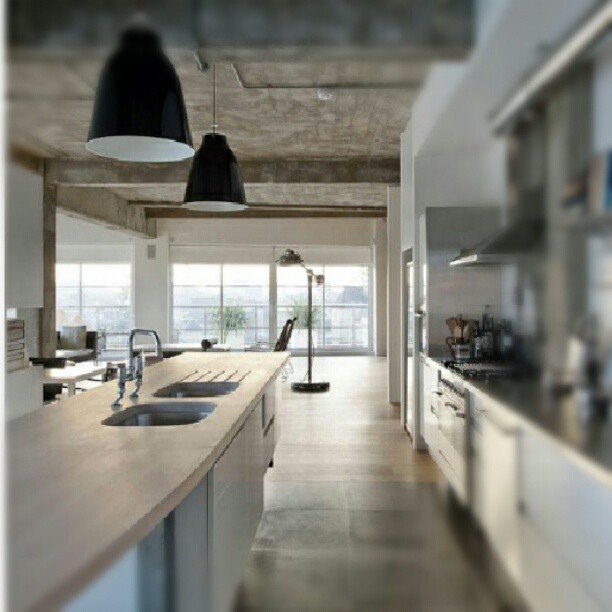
[86,0,193,163]
[182,63,249,213]
[276,248,329,391]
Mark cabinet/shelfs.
[174,395,265,611]
[417,362,469,511]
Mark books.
[6,318,33,372]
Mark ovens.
[436,379,472,464]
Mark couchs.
[57,326,97,362]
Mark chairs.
[127,329,163,372]
[257,316,297,372]
[241,319,293,381]
[29,357,66,404]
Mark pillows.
[60,326,87,349]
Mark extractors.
[447,99,551,269]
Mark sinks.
[157,382,238,396]
[114,405,210,424]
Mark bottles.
[482,305,494,360]
[494,317,504,363]
[504,318,516,363]
[471,319,483,362]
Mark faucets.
[117,328,163,405]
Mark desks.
[43,359,127,396]
[56,349,92,358]
